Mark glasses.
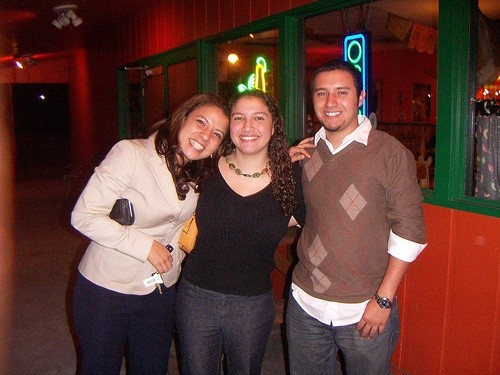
[152,245,173,276]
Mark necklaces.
[225,154,274,178]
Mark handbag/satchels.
[178,210,198,254]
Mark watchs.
[374,291,395,309]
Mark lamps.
[52,4,83,29]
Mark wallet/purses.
[109,199,135,226]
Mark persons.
[67,93,316,375]
[172,89,304,375]
[287,59,427,375]
